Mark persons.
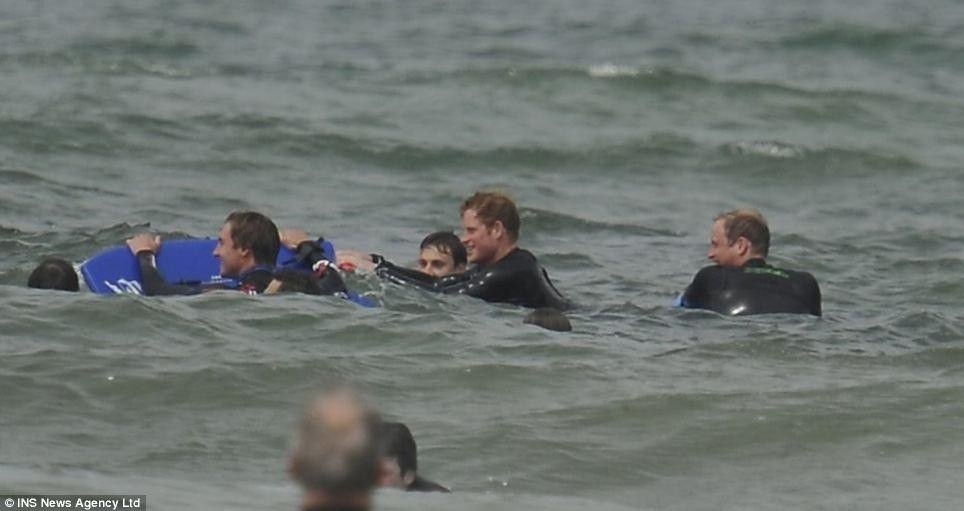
[27,259,78,294]
[522,306,572,333]
[370,422,450,494]
[126,211,377,308]
[676,207,822,316]
[287,388,379,511]
[337,192,567,310]
[419,230,466,278]
